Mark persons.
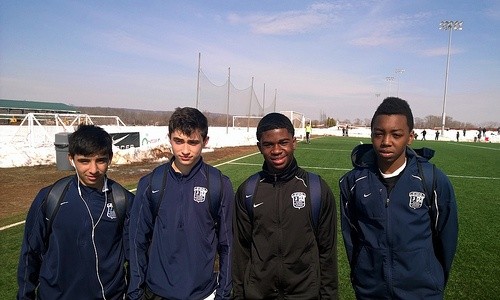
[129,106,232,300]
[232,113,339,300]
[305,123,311,144]
[17,125,134,300]
[341,126,349,137]
[413,127,500,143]
[339,97,459,300]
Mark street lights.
[375,94,381,108]
[384,75,395,97]
[394,69,406,98]
[437,21,464,137]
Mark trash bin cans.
[54,132,76,170]
